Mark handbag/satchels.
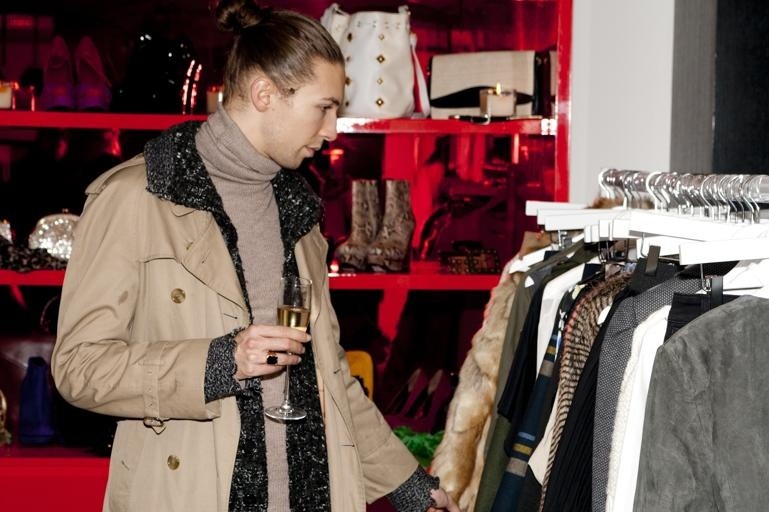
[429,49,549,118]
[319,3,431,121]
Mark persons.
[49,2,461,511]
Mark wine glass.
[262,273,311,422]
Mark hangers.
[525,164,769,311]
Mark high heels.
[406,369,449,431]
[383,368,427,426]
[39,38,77,111]
[72,36,112,111]
[19,356,56,445]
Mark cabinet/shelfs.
[0,0,576,512]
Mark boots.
[337,182,382,269]
[366,179,415,272]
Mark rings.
[265,351,280,367]
